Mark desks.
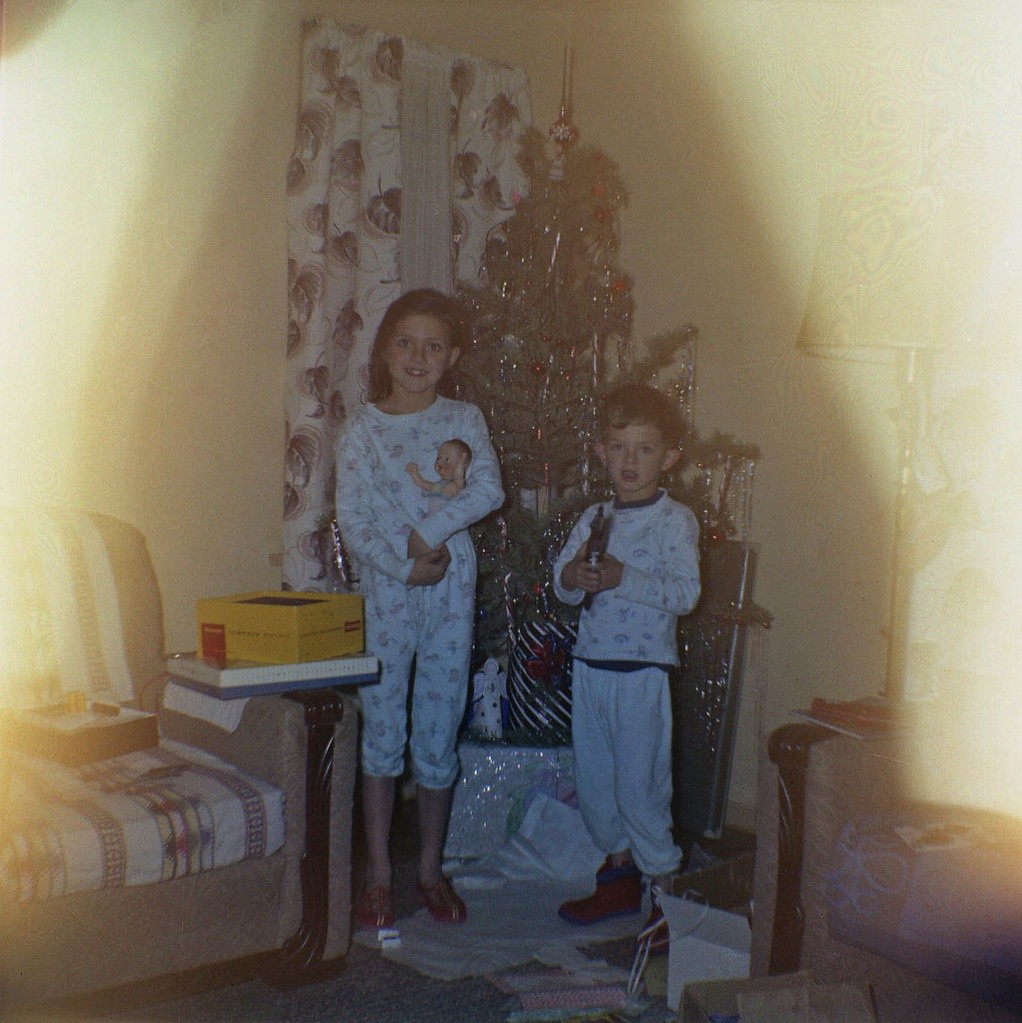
[769,695,1020,977]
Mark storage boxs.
[448,740,582,858]
[11,703,159,767]
[661,847,758,1012]
[677,964,877,1022]
[168,654,380,700]
[194,590,365,662]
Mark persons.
[405,439,471,514]
[552,384,700,958]
[337,287,505,927]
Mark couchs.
[0,501,352,1023]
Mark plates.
[793,697,901,740]
[895,819,995,853]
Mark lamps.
[796,180,984,727]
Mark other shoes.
[636,904,669,956]
[358,880,394,929]
[416,872,466,923]
[558,855,642,926]
